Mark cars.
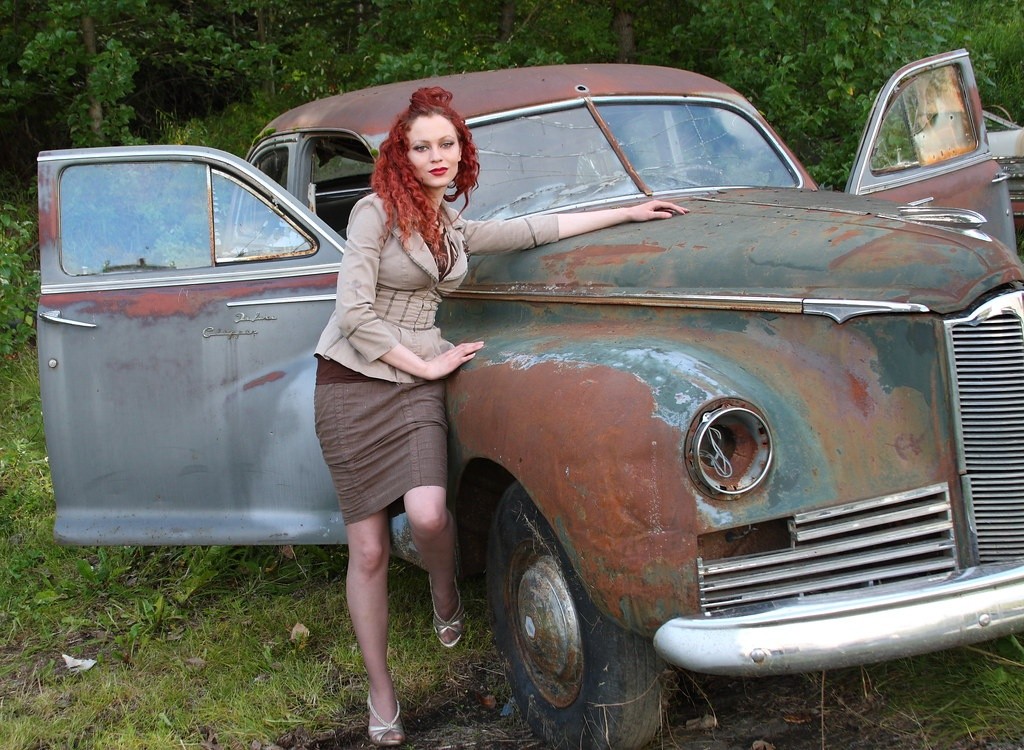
[38,47,1024,750]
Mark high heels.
[367,688,406,746]
[429,573,463,649]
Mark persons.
[315,85,689,746]
